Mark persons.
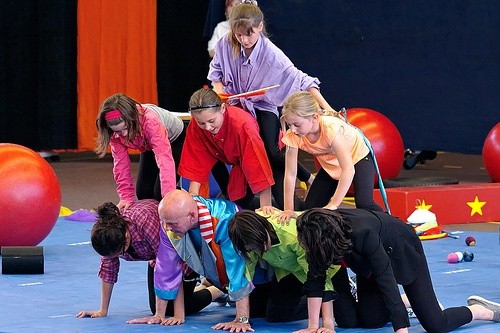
[207,0,343,187]
[178,87,305,212]
[95,94,189,211]
[273,91,383,226]
[77,189,500,333]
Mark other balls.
[465,236,475,246]
[0,143,61,246]
[482,122,500,183]
[338,108,404,197]
[448,251,475,262]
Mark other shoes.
[203,279,236,307]
[467,295,500,322]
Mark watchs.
[237,317,250,323]
